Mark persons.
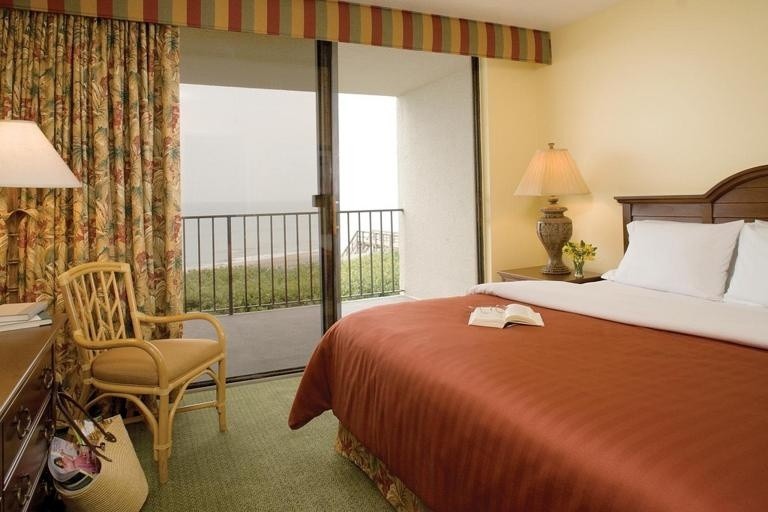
[53,449,74,470]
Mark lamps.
[0,117,85,303]
[513,141,594,276]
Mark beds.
[283,158,767,512]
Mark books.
[468,304,545,329]
[47,436,94,491]
[0,311,53,331]
[1,302,48,321]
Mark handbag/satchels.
[43,414,150,512]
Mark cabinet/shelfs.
[0,315,76,511]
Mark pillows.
[600,215,768,310]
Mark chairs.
[53,257,229,486]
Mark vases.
[573,257,585,278]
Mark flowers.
[561,239,596,263]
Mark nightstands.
[496,265,605,286]
